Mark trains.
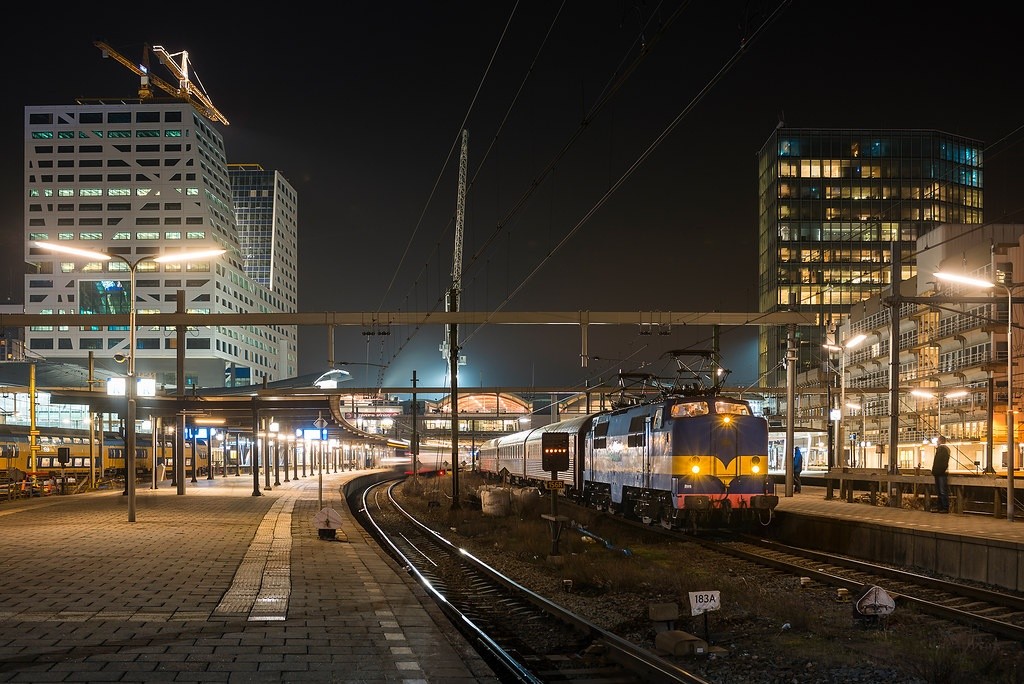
[0,422,224,501]
[474,394,780,534]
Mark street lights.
[932,271,1014,522]
[34,240,227,522]
[910,388,969,438]
[822,334,868,468]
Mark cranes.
[440,129,467,367]
[95,40,229,125]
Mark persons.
[793,446,802,494]
[930,435,951,514]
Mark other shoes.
[939,509,949,513]
[930,508,939,512]
